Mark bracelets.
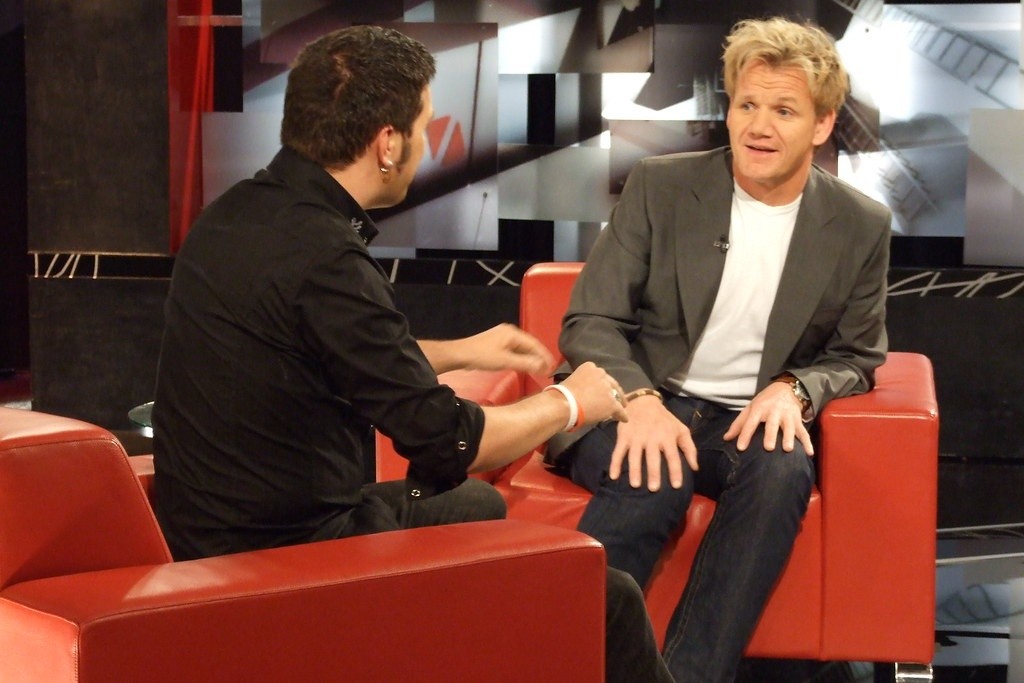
[550,388,584,432]
[625,389,664,404]
[544,385,579,432]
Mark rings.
[612,389,620,398]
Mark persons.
[541,19,890,683]
[153,25,677,683]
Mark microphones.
[719,235,727,253]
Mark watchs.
[774,377,811,412]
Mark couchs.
[0,401,606,683]
[378,264,938,683]
